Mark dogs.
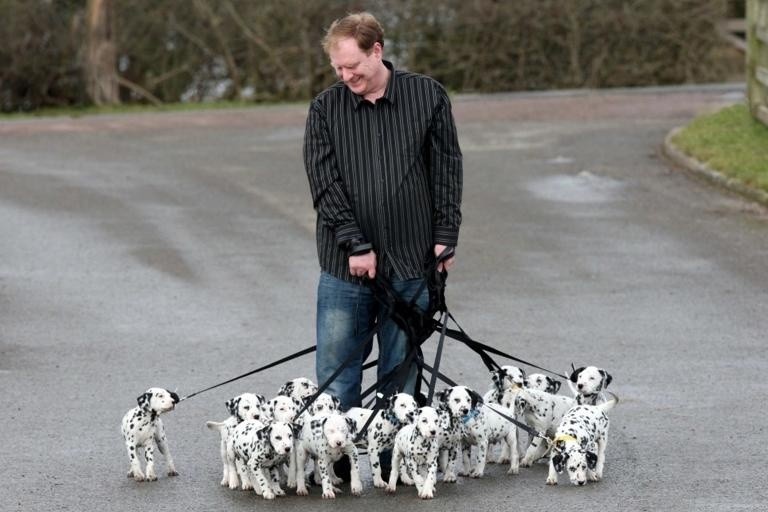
[206,365,619,500]
[121,386,179,482]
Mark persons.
[301,10,464,490]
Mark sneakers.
[308,455,350,486]
[379,450,404,486]
